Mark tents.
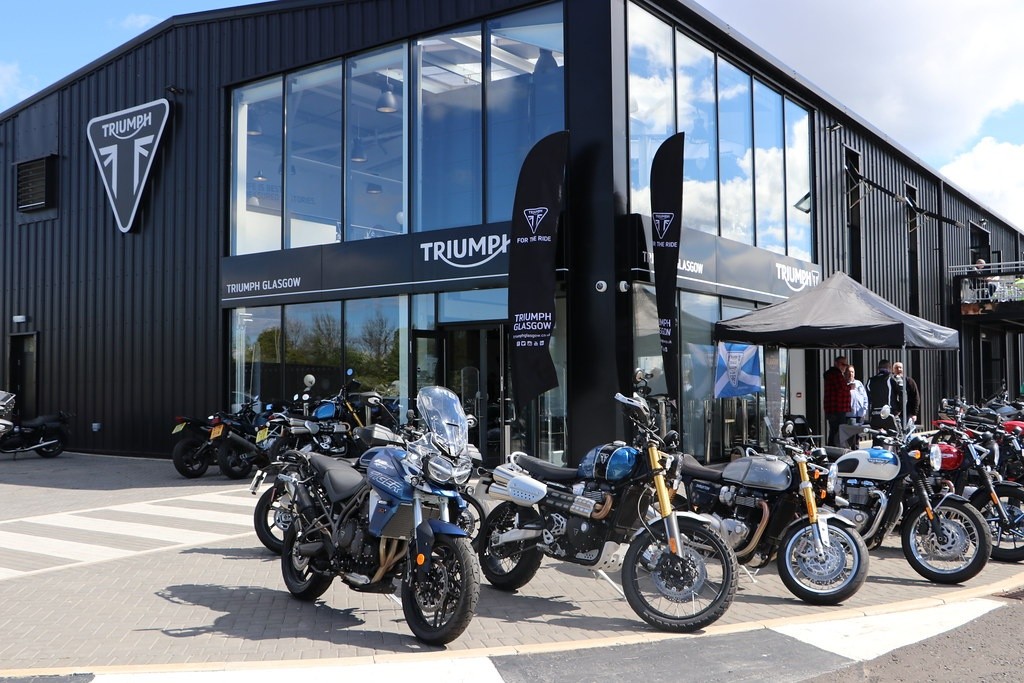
[705,271,961,462]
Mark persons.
[893,362,920,435]
[866,360,908,447]
[970,259,996,298]
[824,356,868,450]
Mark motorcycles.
[165,375,356,482]
[625,416,871,604]
[0,391,70,459]
[744,403,994,585]
[277,385,485,650]
[480,369,740,634]
[820,382,1024,564]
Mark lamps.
[979,219,989,225]
[827,123,843,132]
